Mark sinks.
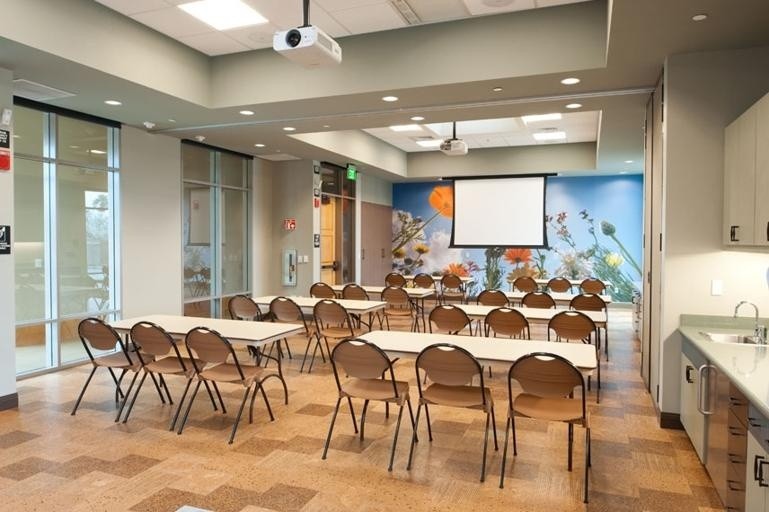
[705,332,759,345]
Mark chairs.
[485,307,531,377]
[314,299,366,365]
[521,291,558,309]
[579,277,607,295]
[512,276,539,308]
[123,321,208,432]
[439,275,468,306]
[406,342,499,482]
[378,286,424,333]
[385,272,417,312]
[270,294,327,365]
[177,326,275,445]
[568,291,609,363]
[321,334,419,472]
[70,316,168,422]
[500,351,592,503]
[341,283,385,332]
[423,302,472,384]
[414,273,443,311]
[546,277,573,294]
[547,309,601,406]
[227,293,286,361]
[474,290,510,339]
[309,281,347,329]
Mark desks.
[249,295,388,339]
[344,329,598,473]
[507,278,611,295]
[331,285,436,332]
[498,291,610,323]
[402,274,475,303]
[493,289,611,311]
[99,314,305,424]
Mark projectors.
[440,141,468,156]
[273,27,342,68]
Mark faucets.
[733,300,759,336]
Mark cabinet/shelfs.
[361,200,393,286]
[680,341,709,467]
[745,400,769,512]
[722,93,769,246]
[640,67,666,426]
[632,290,642,338]
[706,362,748,512]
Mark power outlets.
[712,278,723,297]
